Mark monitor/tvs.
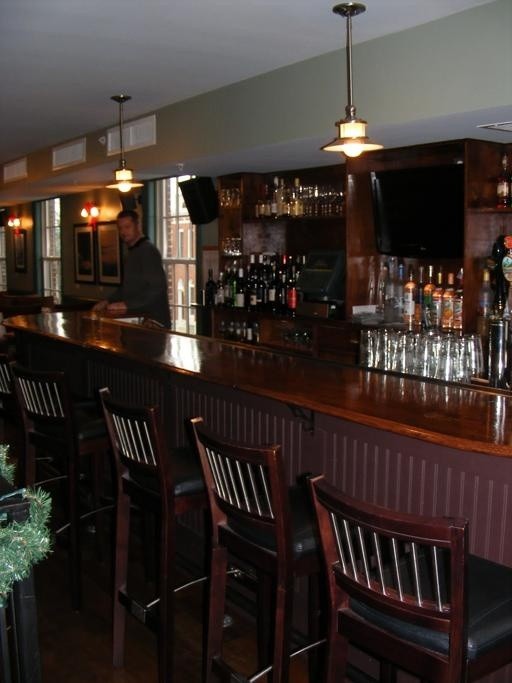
[370,164,464,259]
[295,250,345,302]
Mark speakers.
[178,176,218,225]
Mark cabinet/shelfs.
[192,171,357,357]
[464,153,510,392]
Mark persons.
[118,325,167,359]
[93,208,172,329]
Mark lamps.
[106,86,144,196]
[320,2,387,161]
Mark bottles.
[281,328,311,348]
[221,237,240,256]
[204,254,299,311]
[220,187,242,209]
[218,311,259,346]
[214,342,299,372]
[489,227,511,320]
[256,174,302,217]
[494,154,510,208]
[378,257,492,337]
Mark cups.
[358,328,485,387]
[363,371,478,414]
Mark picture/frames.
[13,228,28,276]
[95,218,124,286]
[73,220,97,285]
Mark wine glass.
[305,184,346,217]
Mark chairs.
[89,380,274,681]
[192,411,338,680]
[9,368,164,611]
[304,462,511,677]
[1,351,16,413]
